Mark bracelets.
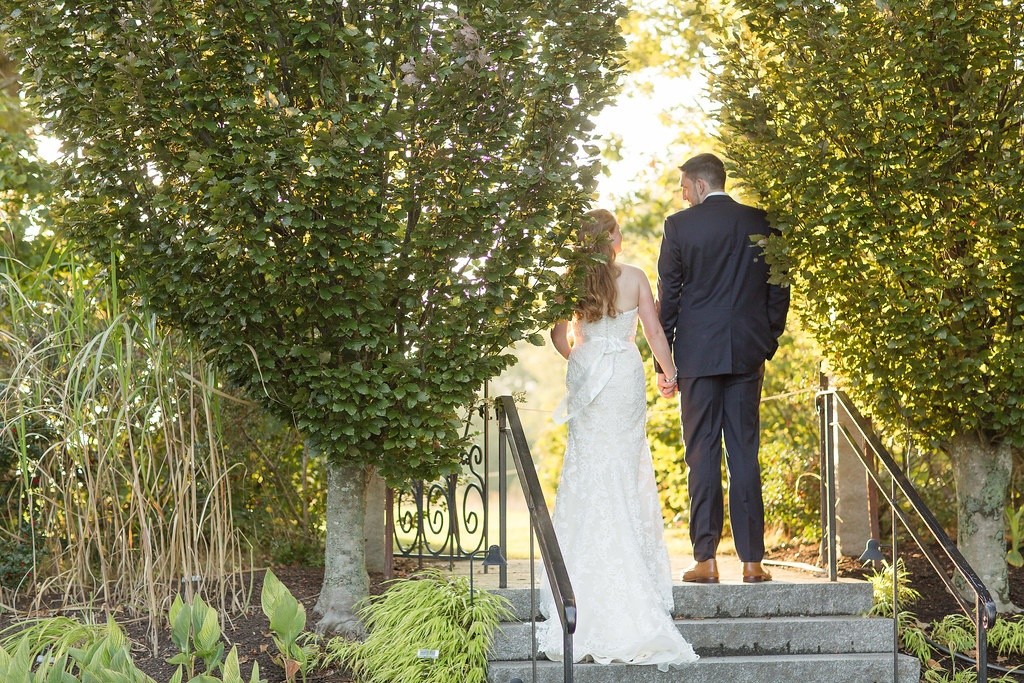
[665,365,681,387]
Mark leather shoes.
[682,559,720,584]
[742,562,772,582]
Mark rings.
[661,388,666,392]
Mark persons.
[533,206,702,668]
[652,151,794,585]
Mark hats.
[868,540,882,549]
